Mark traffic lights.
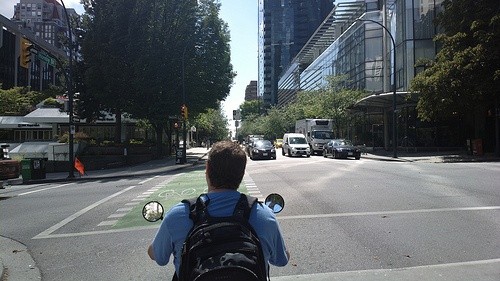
[20,37,34,70]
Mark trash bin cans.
[465,136,484,156]
[20,157,48,180]
[175,144,185,164]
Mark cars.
[250,139,275,160]
[273,139,283,149]
[322,139,361,161]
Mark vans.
[281,134,312,159]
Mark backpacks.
[178,194,270,281]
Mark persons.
[147,141,290,281]
[266,195,282,212]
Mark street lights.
[356,17,398,159]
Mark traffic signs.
[70,116,81,125]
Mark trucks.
[294,118,334,155]
[245,135,264,152]
